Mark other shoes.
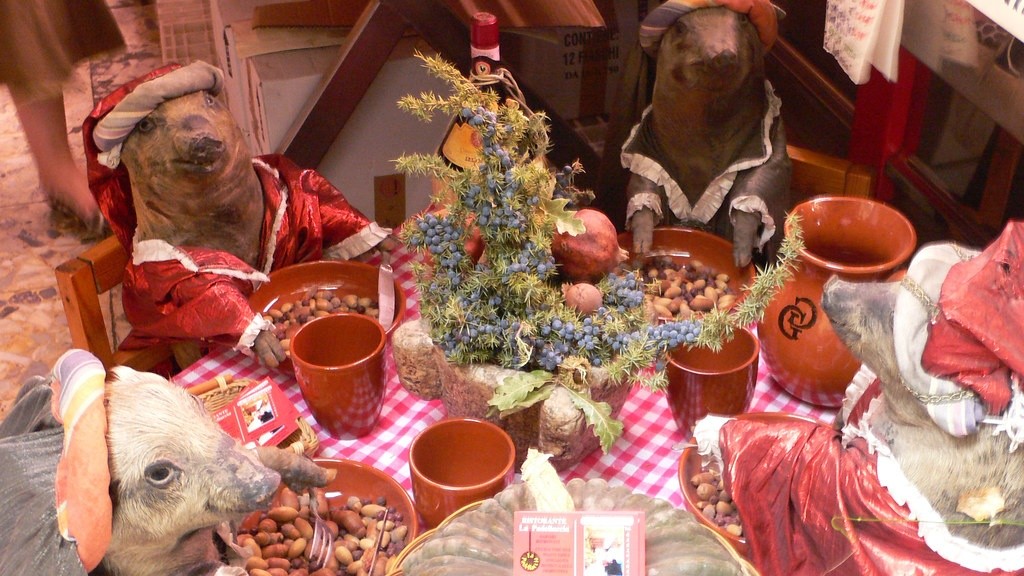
[42,169,114,239]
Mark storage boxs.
[494,9,631,123]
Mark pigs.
[689,219,1024,576]
[0,348,337,576]
[83,60,401,381]
[626,0,794,276]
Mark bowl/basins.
[678,412,834,561]
[612,227,756,324]
[247,260,407,377]
[236,459,418,555]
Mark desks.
[167,220,837,516]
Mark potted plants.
[393,50,807,473]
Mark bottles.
[757,197,916,406]
[433,11,547,217]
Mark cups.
[664,323,761,442]
[409,417,516,528]
[290,312,387,440]
